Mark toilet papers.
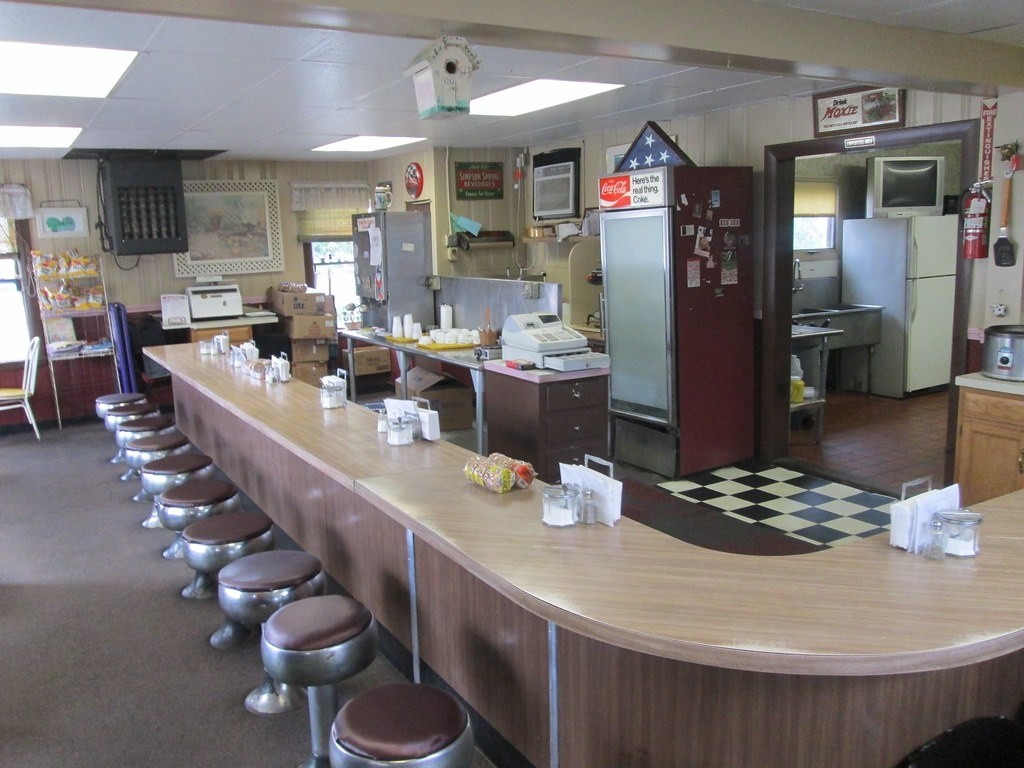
[440,305,452,330]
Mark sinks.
[820,304,866,310]
[791,314,798,315]
[799,309,831,314]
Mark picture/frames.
[812,86,905,138]
[171,180,285,279]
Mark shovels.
[994,171,1016,267]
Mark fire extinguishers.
[959,180,990,258]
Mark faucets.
[792,259,803,294]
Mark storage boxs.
[343,346,391,376]
[395,366,475,430]
[266,287,337,388]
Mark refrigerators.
[842,213,961,398]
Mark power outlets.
[447,246,458,260]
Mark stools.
[96,392,472,768]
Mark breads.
[465,454,534,493]
[279,282,307,293]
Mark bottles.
[320,384,325,404]
[264,366,276,385]
[373,408,388,433]
[922,520,948,562]
[579,488,600,525]
[209,342,217,356]
[229,351,241,368]
[790,375,805,404]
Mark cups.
[391,313,423,339]
[375,187,392,211]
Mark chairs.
[0,335,43,440]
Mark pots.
[980,325,1024,383]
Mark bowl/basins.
[419,328,478,344]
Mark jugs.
[587,310,600,328]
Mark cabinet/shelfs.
[178,326,253,351]
[484,361,610,486]
[790,324,843,440]
[953,387,1024,507]
[33,254,122,430]
